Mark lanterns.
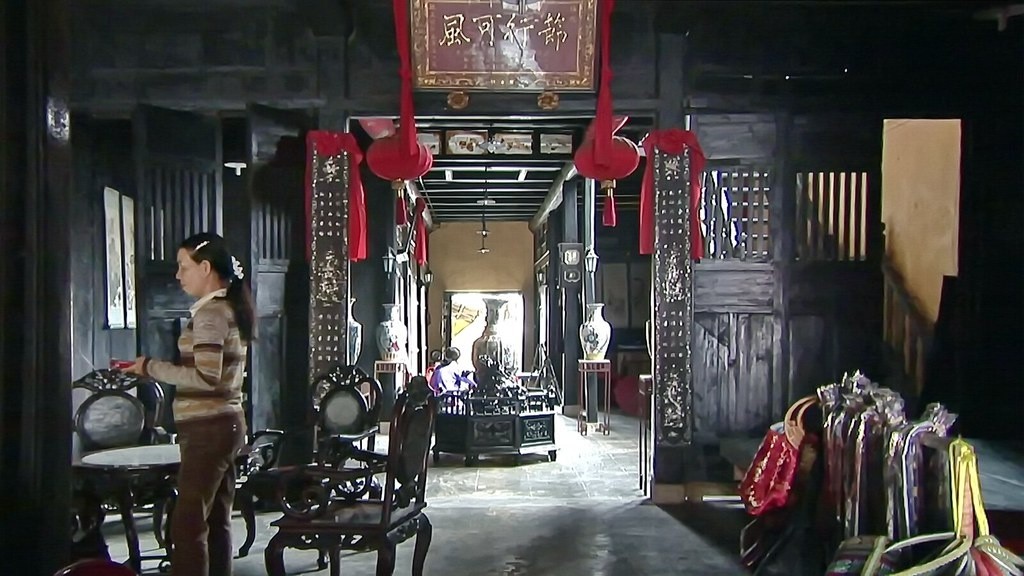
[367,135,433,224]
[574,136,640,227]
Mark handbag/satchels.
[739,366,1023,576]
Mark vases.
[349,297,361,365]
[375,303,407,360]
[579,304,612,361]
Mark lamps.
[537,265,546,286]
[382,245,397,281]
[476,164,496,206]
[585,244,600,272]
[478,213,491,236]
[476,235,491,254]
[417,269,435,292]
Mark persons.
[428,347,464,407]
[122,232,259,576]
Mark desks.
[77,443,245,549]
[372,360,405,430]
[640,374,653,496]
[577,358,611,436]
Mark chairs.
[68,368,177,549]
[262,375,436,576]
[40,465,142,576]
[263,365,383,499]
